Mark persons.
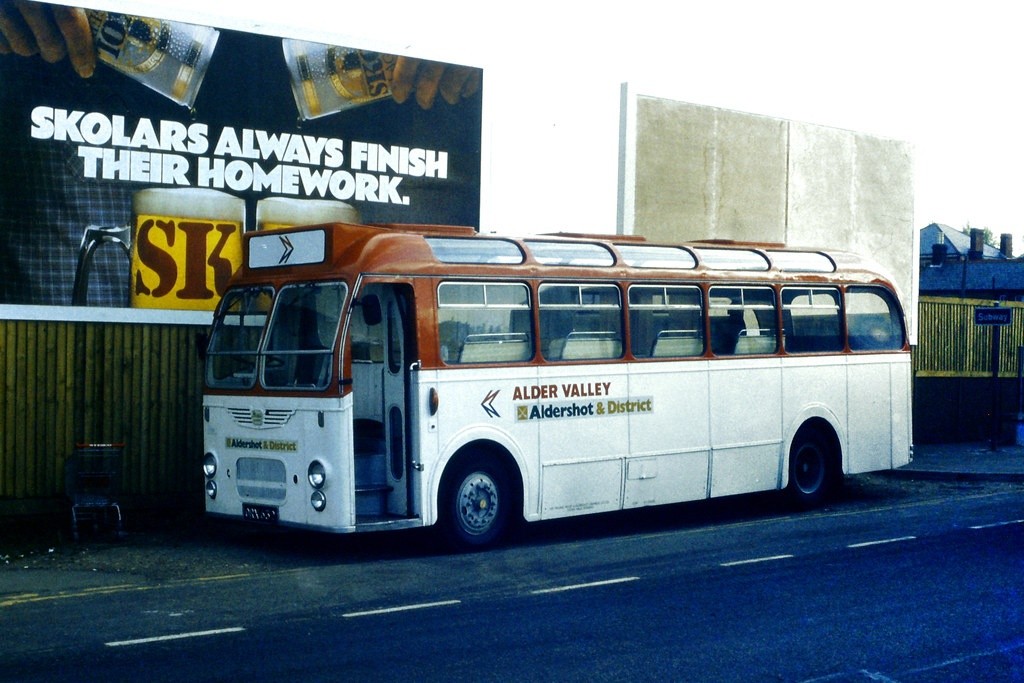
[1,0,482,309]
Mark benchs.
[458,333,532,362]
[549,330,622,360]
[734,329,786,355]
[649,330,704,357]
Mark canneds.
[83,8,220,109]
[282,38,418,121]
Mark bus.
[193,220,916,553]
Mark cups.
[72,212,244,310]
[256,222,299,306]
